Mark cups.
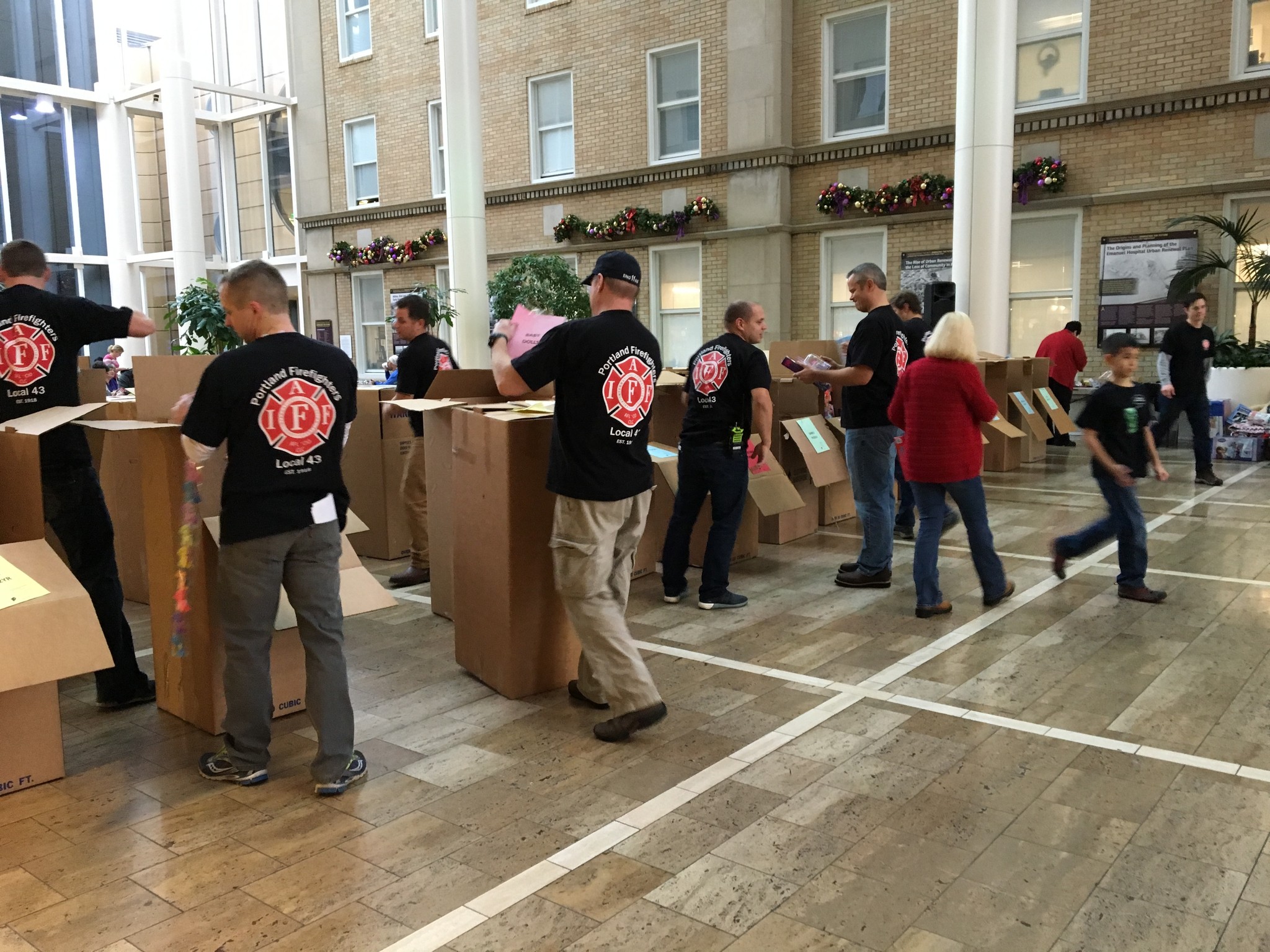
[804,352,832,372]
[382,362,389,368]
[780,356,831,391]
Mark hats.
[581,251,641,288]
[389,355,399,365]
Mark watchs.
[487,332,509,350]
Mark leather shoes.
[389,567,430,583]
[834,570,891,588]
[838,563,860,573]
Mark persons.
[487,248,671,744]
[105,364,120,392]
[1143,291,1226,488]
[1049,331,1170,604]
[103,344,129,383]
[1033,319,1089,448]
[885,309,1016,620]
[92,360,112,396]
[379,293,459,588]
[0,238,163,714]
[117,367,135,396]
[657,296,775,614]
[791,261,911,591]
[370,355,399,385]
[838,289,962,542]
[170,257,371,797]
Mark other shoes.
[915,600,952,618]
[568,679,609,710]
[1062,439,1076,447]
[1118,584,1167,602]
[593,703,668,741]
[1052,537,1066,579]
[984,579,1015,605]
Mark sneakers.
[664,576,688,603]
[198,744,268,785]
[941,511,959,536]
[315,750,367,793]
[698,590,748,610]
[95,680,156,707]
[1195,470,1223,486]
[893,525,914,539]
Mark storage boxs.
[0,339,1078,801]
[1207,397,1269,459]
